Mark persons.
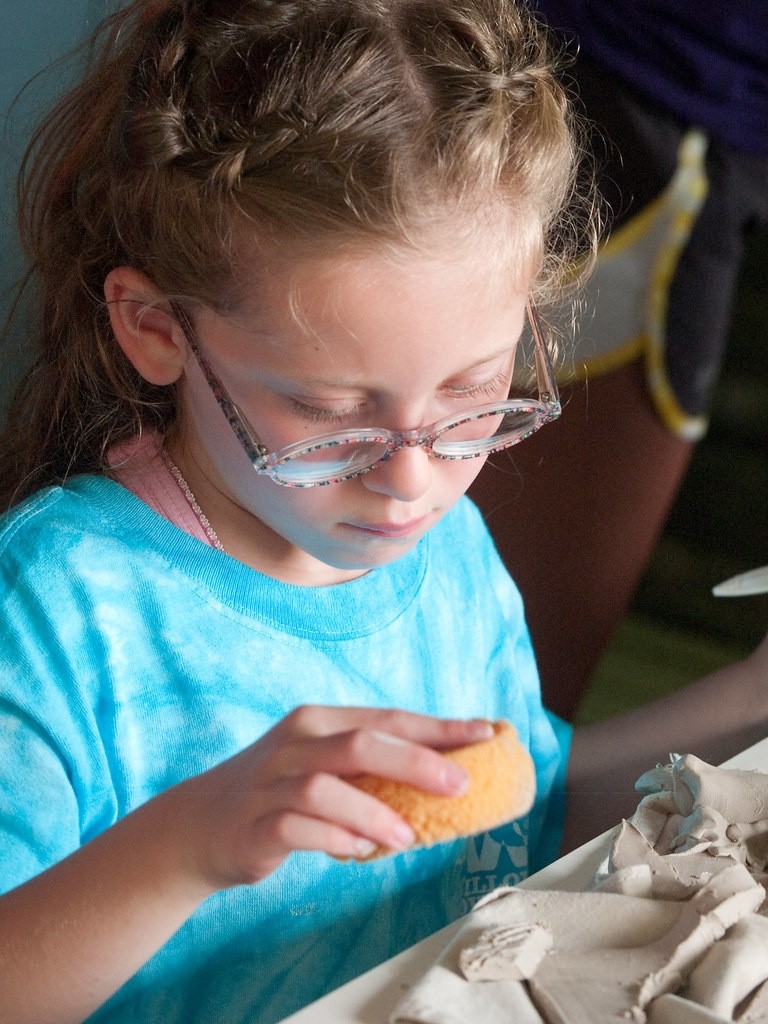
[2,2,767,1024]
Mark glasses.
[158,282,564,489]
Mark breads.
[330,719,538,862]
